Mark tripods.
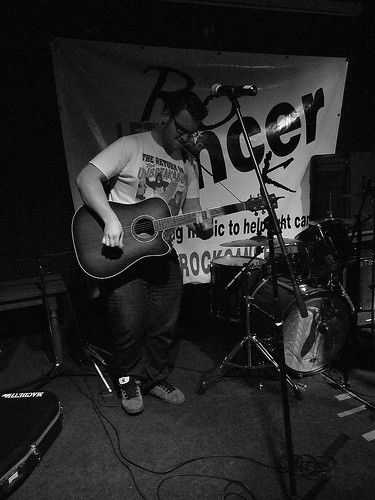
[197,245,306,397]
[5,247,115,394]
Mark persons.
[76,89,214,414]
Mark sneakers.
[118,375,144,414]
[148,379,185,405]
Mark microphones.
[210,84,259,98]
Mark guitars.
[71,196,278,280]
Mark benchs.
[0,273,68,364]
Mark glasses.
[172,110,198,138]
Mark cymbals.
[220,232,300,249]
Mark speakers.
[343,258,375,327]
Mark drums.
[250,274,340,377]
[292,220,357,278]
[208,256,275,325]
